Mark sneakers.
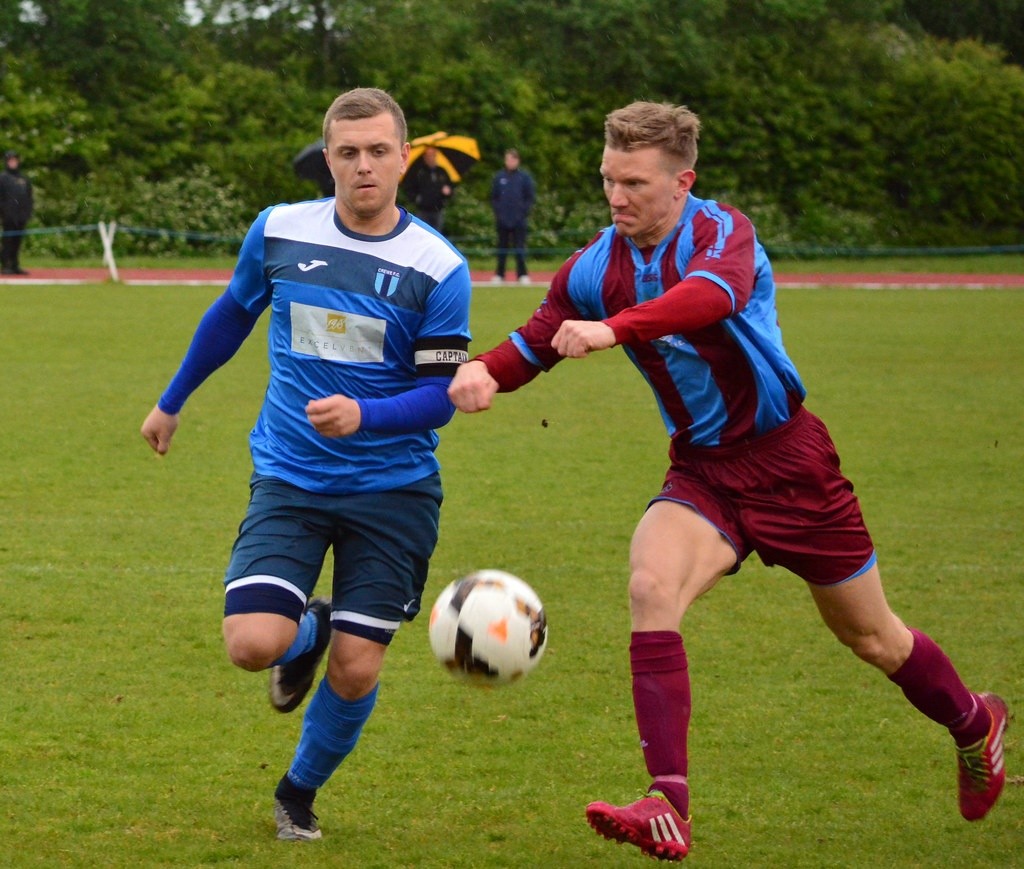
[585,790,692,860]
[270,597,332,712]
[956,693,1008,822]
[272,771,321,840]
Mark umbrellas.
[293,139,326,181]
[399,131,480,183]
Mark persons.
[140,87,473,838]
[488,147,536,285]
[0,152,34,276]
[448,102,1008,862]
[401,146,452,234]
[317,162,336,198]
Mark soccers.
[429,567,548,686]
